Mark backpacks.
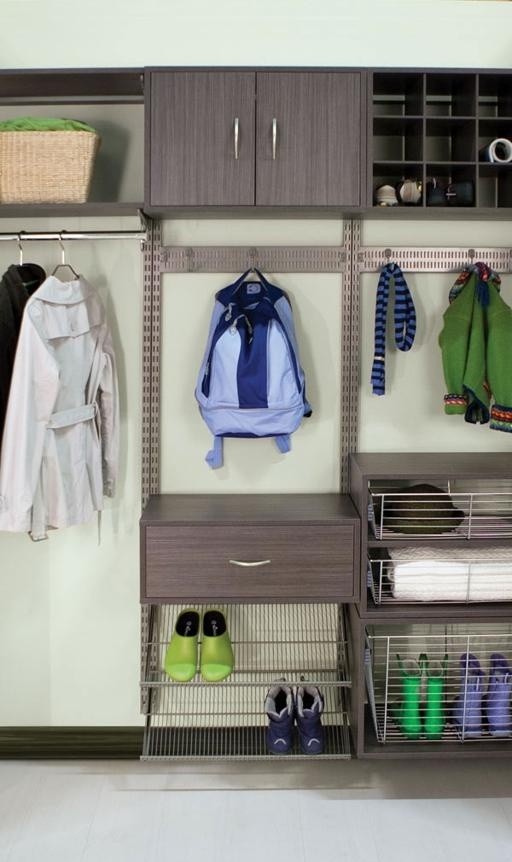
[192,267,313,469]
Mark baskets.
[1,130,103,207]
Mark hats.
[376,484,466,534]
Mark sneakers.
[164,607,200,682]
[200,608,234,682]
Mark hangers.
[52,231,80,279]
[12,231,39,286]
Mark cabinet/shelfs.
[144,67,367,220]
[139,493,358,760]
[353,451,512,759]
[367,68,512,221]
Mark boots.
[389,653,424,740]
[263,678,295,755]
[486,654,511,737]
[295,677,326,755]
[451,653,486,736]
[424,654,448,740]
